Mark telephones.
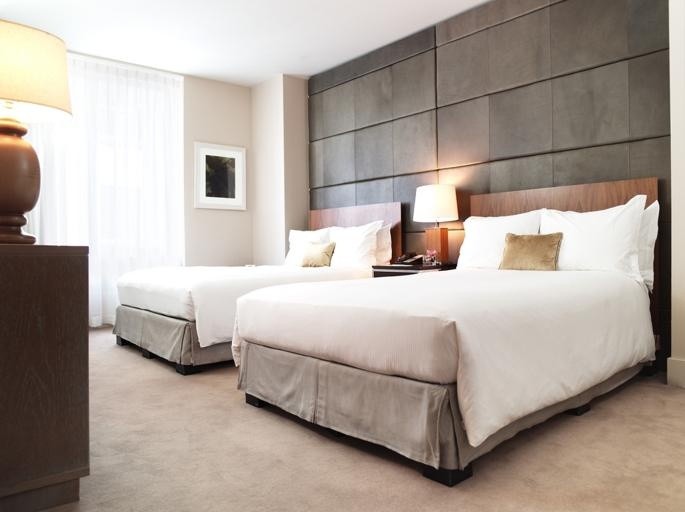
[394,252,424,265]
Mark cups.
[422,255,437,266]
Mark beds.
[111,200,412,375]
[228,176,661,488]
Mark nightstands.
[371,263,457,278]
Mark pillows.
[539,194,647,283]
[497,231,563,271]
[283,228,335,267]
[455,210,540,272]
[330,221,384,273]
[376,222,394,265]
[300,240,336,270]
[636,199,659,292]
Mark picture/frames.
[193,142,247,211]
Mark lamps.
[1,19,75,244]
[411,183,460,266]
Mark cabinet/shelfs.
[0,244,91,512]
